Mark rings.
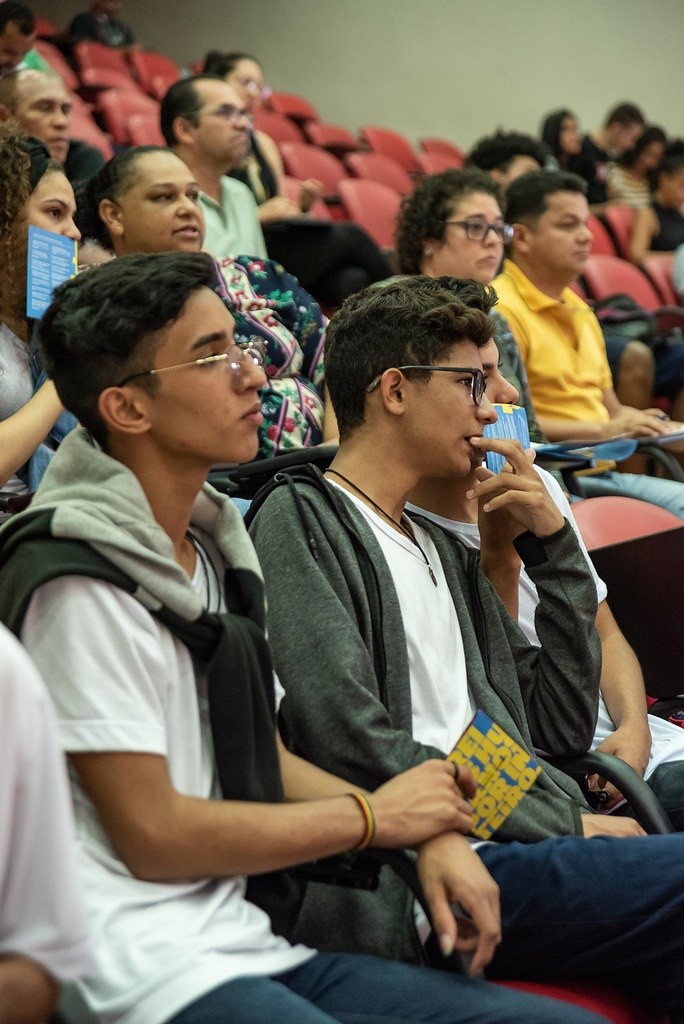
[452,761,459,779]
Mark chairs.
[22,16,683,1024]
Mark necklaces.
[188,532,221,612]
[325,468,437,588]
[188,536,209,612]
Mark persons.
[0,0,683,1024]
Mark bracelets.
[346,792,375,851]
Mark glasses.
[116,338,266,388]
[225,75,272,99]
[444,218,513,246]
[180,107,255,127]
[364,365,488,406]
